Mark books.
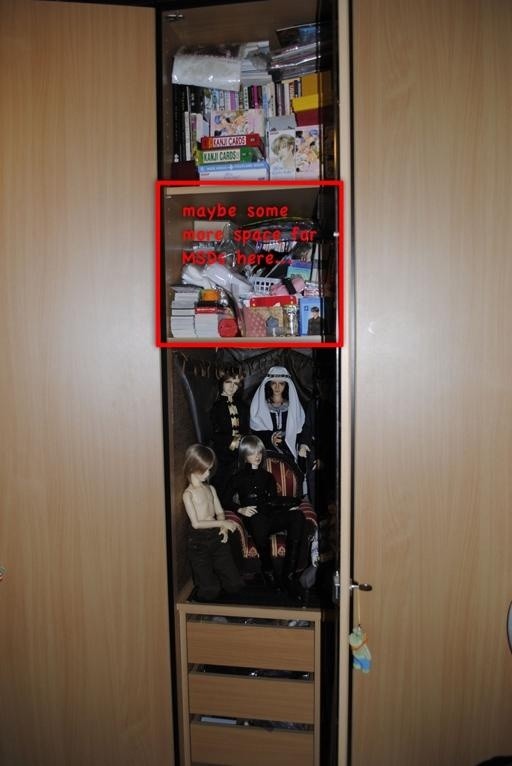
[170,22,324,187]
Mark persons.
[183,365,321,628]
[252,240,311,278]
[307,306,324,336]
